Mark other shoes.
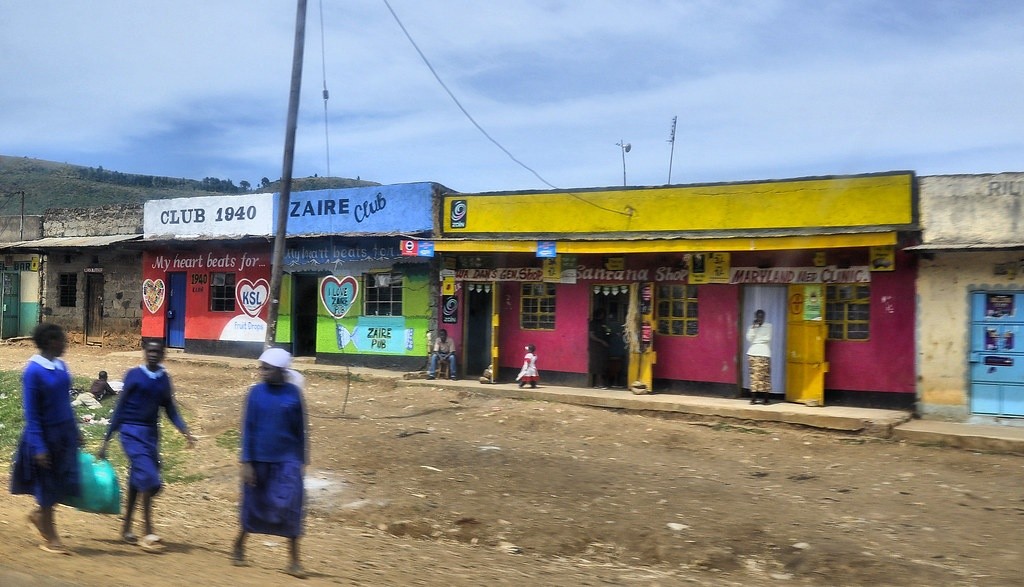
[233,546,247,566]
[596,384,605,387]
[452,377,457,381]
[427,376,434,380]
[287,562,308,578]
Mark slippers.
[38,544,72,555]
[120,533,140,544]
[139,534,167,552]
[28,515,50,542]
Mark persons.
[515,344,540,387]
[590,307,612,389]
[10,321,85,556]
[744,309,772,404]
[95,337,198,552]
[233,348,313,579]
[426,329,458,381]
[70,371,116,409]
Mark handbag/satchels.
[55,450,120,514]
